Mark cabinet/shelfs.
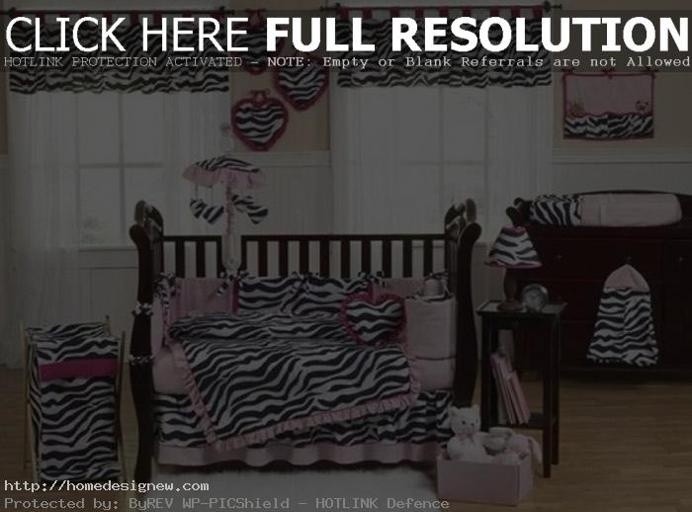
[507,190,692,381]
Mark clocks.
[522,284,549,311]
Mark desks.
[475,299,568,477]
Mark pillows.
[340,294,406,345]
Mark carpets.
[146,469,439,512]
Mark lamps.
[485,227,542,312]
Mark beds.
[128,199,481,485]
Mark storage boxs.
[436,452,533,507]
